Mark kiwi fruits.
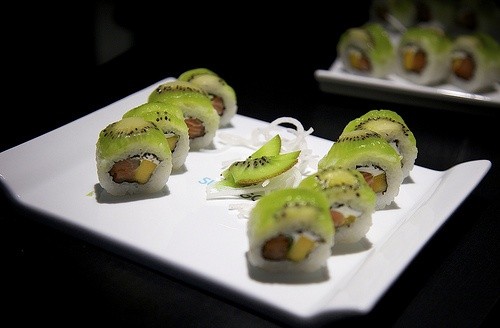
[218,135,301,188]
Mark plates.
[314,31,499,115]
[0,75,492,322]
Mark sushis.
[248,110,418,270]
[96,68,237,197]
[338,8,500,93]
